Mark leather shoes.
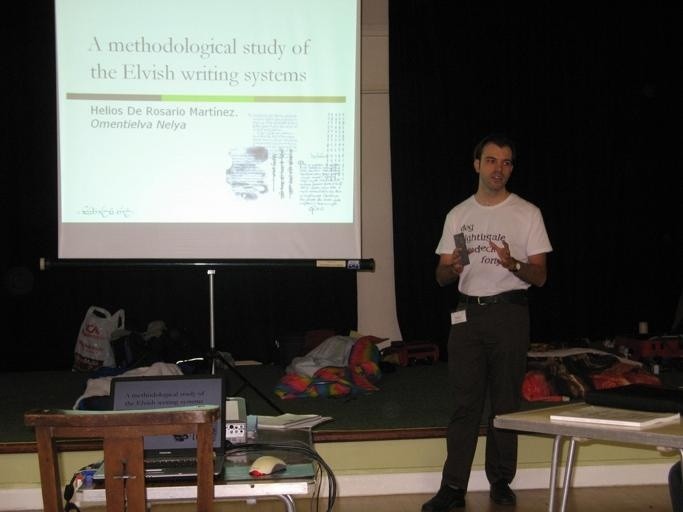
[461,294,529,307]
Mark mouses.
[248,454,290,478]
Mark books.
[549,405,680,428]
[249,412,335,431]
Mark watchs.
[507,259,522,273]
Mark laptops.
[90,374,228,483]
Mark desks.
[75,423,320,512]
[493,401,683,511]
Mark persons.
[421,135,553,512]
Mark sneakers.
[420,486,467,512]
[489,481,517,506]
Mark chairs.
[23,408,219,512]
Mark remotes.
[454,232,471,265]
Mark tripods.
[159,268,288,421]
